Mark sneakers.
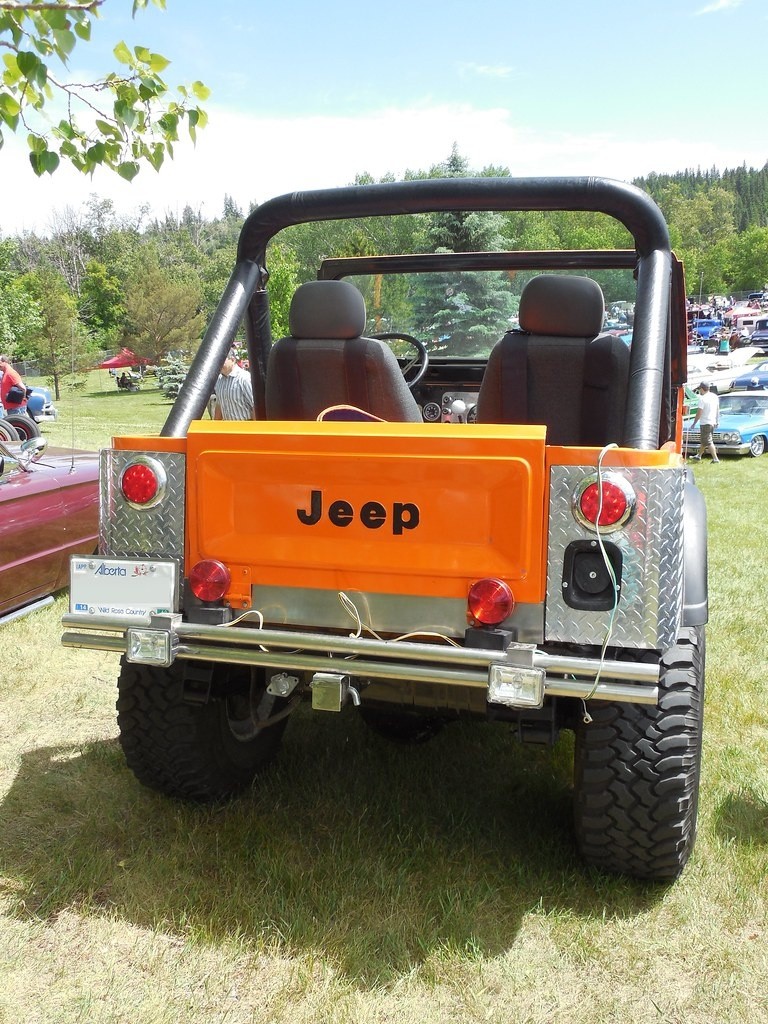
[710,459,719,464]
[689,454,701,462]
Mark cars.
[691,319,720,341]
[679,391,768,458]
[748,319,768,350]
[0,384,59,426]
[725,360,768,395]
[748,292,765,301]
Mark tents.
[721,307,761,318]
[101,348,151,391]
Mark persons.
[604,306,627,321]
[214,348,256,420]
[109,367,117,377]
[686,298,761,326]
[117,372,133,389]
[688,381,720,463]
[0,355,28,416]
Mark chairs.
[116,376,132,394]
[262,280,423,423]
[476,275,630,446]
[740,399,763,414]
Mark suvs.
[48,174,714,885]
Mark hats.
[698,380,709,389]
